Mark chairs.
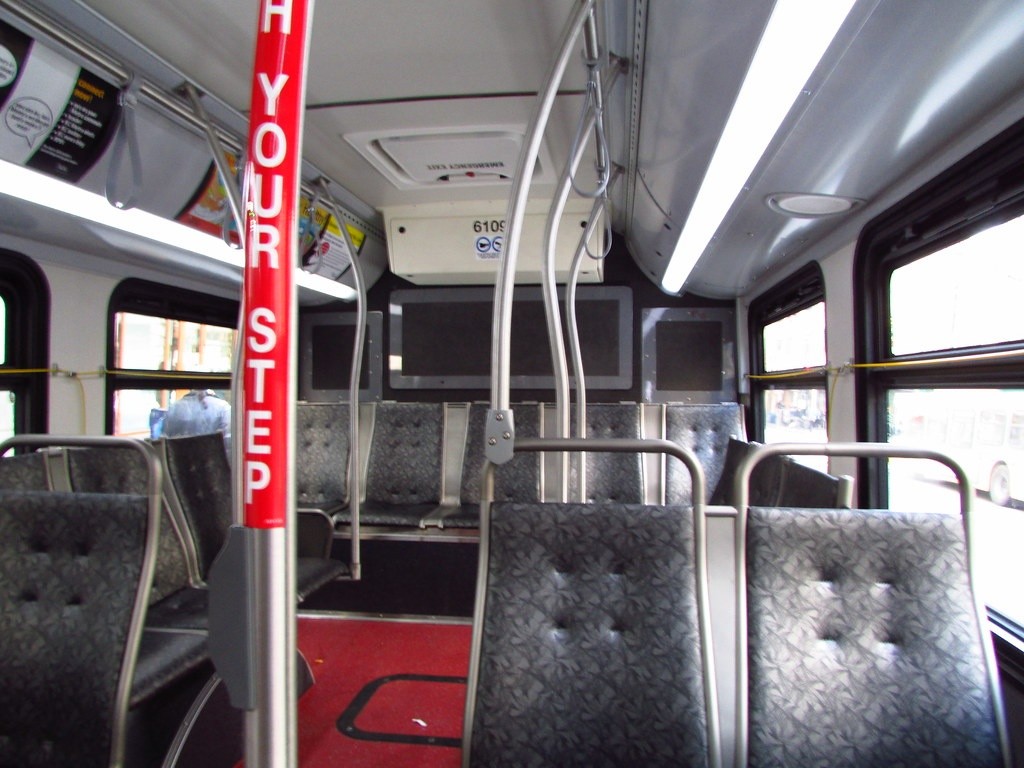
[0,400,1012,768]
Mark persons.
[161,388,232,468]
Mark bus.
[904,387,1024,511]
